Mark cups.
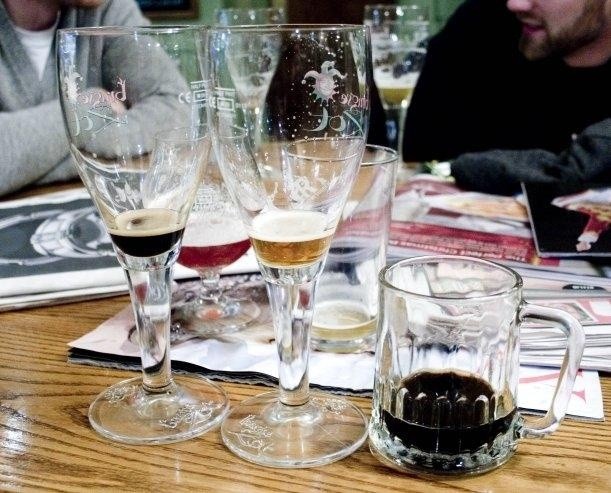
[282,139,405,350]
[368,255,589,477]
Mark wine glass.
[204,23,367,469]
[59,23,231,449]
[144,123,262,338]
[368,5,434,170]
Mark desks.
[0,143,611,492]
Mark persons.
[1,1,201,197]
[400,0,611,192]
